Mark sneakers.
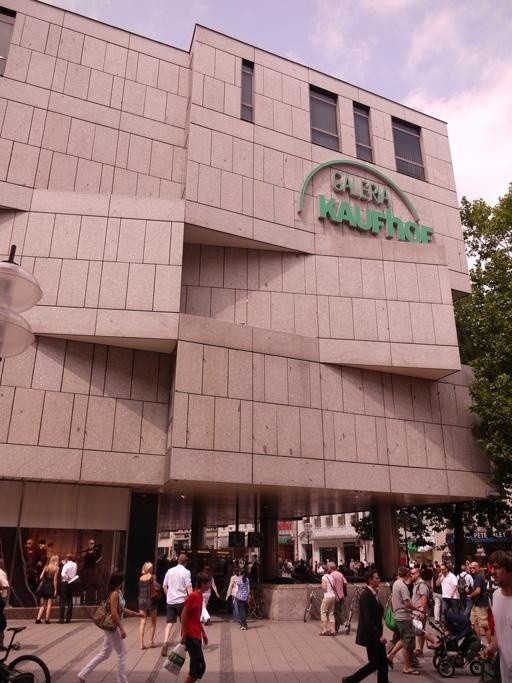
[344,622,351,636]
[240,625,247,630]
[161,642,168,657]
[413,649,425,658]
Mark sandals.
[330,630,338,636]
[318,630,330,636]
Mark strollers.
[419,610,484,677]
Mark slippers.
[385,653,394,670]
[402,668,422,675]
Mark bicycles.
[382,580,393,618]
[346,577,369,635]
[0,625,53,683]
[248,587,265,619]
[302,583,347,624]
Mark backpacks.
[457,573,469,594]
[417,582,435,609]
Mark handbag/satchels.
[149,573,163,600]
[383,595,397,632]
[35,566,47,595]
[92,590,123,632]
[334,597,341,608]
[163,643,187,676]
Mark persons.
[137,562,162,650]
[179,572,212,683]
[225,551,512,683]
[15,539,38,605]
[0,568,9,647]
[36,540,46,576]
[76,572,145,683]
[194,565,221,625]
[161,554,194,656]
[79,539,103,605]
[35,555,60,624]
[58,552,78,623]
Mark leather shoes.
[34,617,71,624]
